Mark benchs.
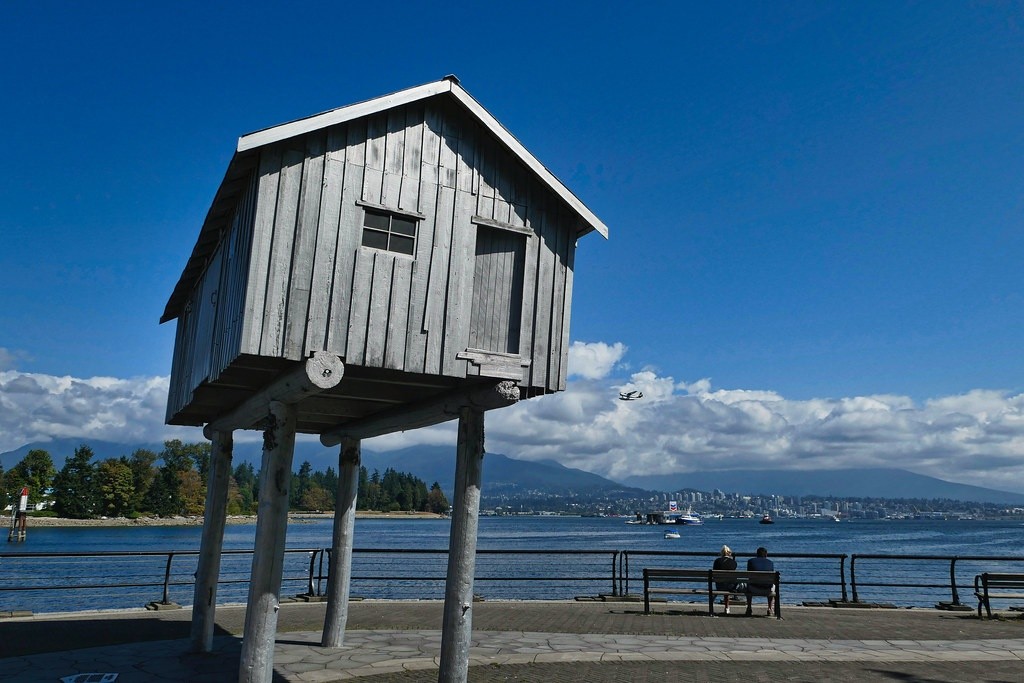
[643,567,782,620]
[973,572,1024,618]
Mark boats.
[677,505,704,525]
[625,518,641,524]
[758,512,774,524]
[664,529,681,539]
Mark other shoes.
[725,608,730,614]
[745,610,753,615]
[767,610,774,615]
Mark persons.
[746,548,776,616]
[713,545,737,614]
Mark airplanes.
[619,391,643,400]
